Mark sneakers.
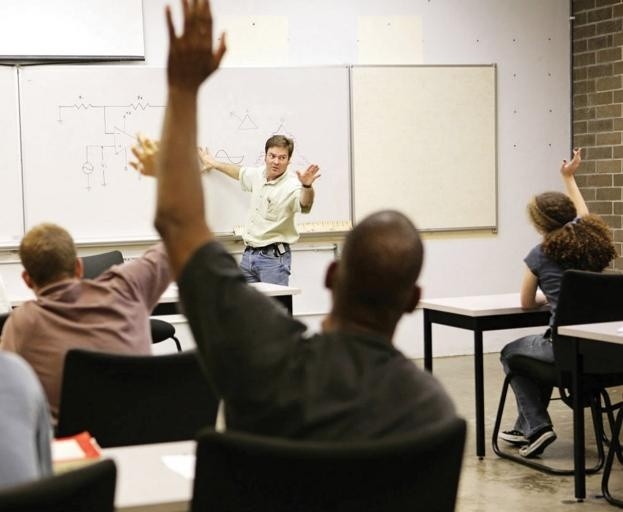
[519,426,557,458]
[498,430,530,445]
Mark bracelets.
[302,184,312,189]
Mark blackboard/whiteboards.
[16,61,355,247]
[0,55,498,254]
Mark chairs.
[0,457,119,512]
[77,248,123,281]
[488,263,623,479]
[51,343,227,448]
[182,416,468,511]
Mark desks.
[0,277,302,334]
[556,312,623,503]
[411,292,551,455]
[102,441,200,511]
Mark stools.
[146,315,183,353]
[597,403,622,509]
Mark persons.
[0,343,53,496]
[499,143,619,461]
[148,0,463,511]
[0,132,178,435]
[195,133,324,286]
